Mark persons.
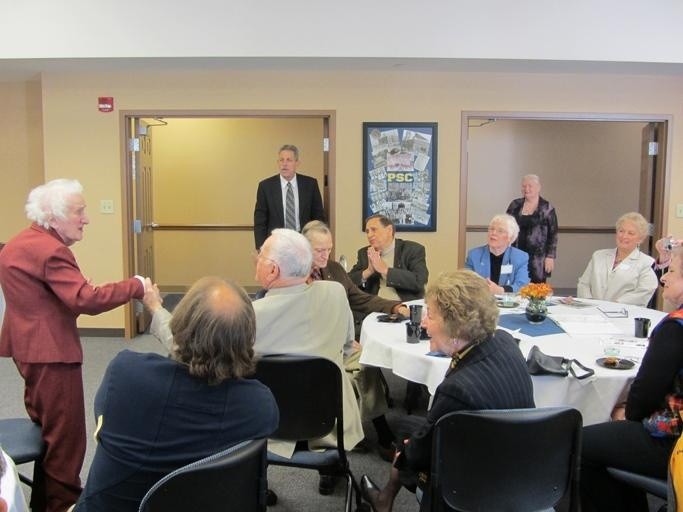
[576,211,658,309]
[252,143,327,255]
[139,225,364,500]
[578,232,683,512]
[357,268,537,511]
[71,272,281,512]
[0,175,163,512]
[504,172,561,286]
[460,211,531,297]
[299,218,412,344]
[346,213,430,304]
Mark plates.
[497,301,518,309]
[595,358,635,369]
[377,314,409,323]
[420,327,431,340]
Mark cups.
[408,305,423,324]
[633,317,650,337]
[407,323,420,343]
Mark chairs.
[582,432,682,512]
[139,436,268,511]
[414,402,583,511]
[0,412,43,485]
[254,352,355,512]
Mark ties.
[285,182,296,231]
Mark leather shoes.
[320,462,349,495]
[361,474,382,512]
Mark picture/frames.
[360,120,440,233]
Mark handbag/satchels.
[643,309,683,440]
[527,346,594,380]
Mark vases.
[525,297,547,322]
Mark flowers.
[519,282,553,301]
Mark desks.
[358,293,667,427]
